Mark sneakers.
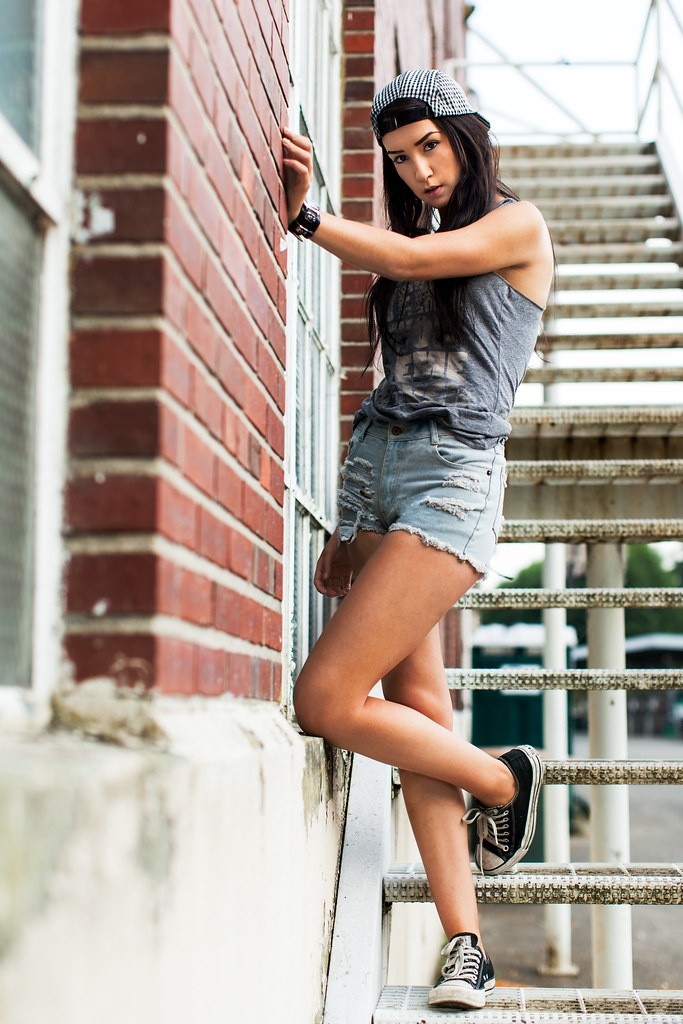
[428,932,496,1010]
[461,743,543,877]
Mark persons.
[282,67,557,1012]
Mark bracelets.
[287,198,322,243]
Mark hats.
[369,68,490,147]
